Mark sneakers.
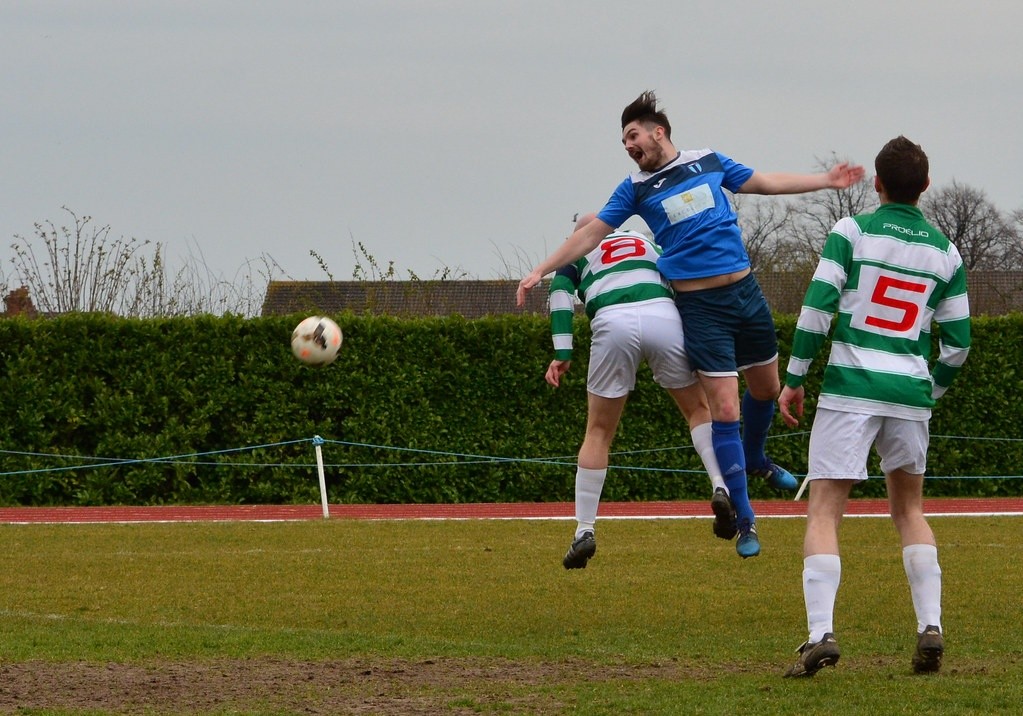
[784,632,839,678]
[745,456,797,489]
[711,488,738,540]
[563,532,595,571]
[910,623,942,674]
[734,517,761,558]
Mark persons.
[778,137,972,679]
[516,89,866,559]
[543,212,737,570]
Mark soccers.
[291,314,344,367]
